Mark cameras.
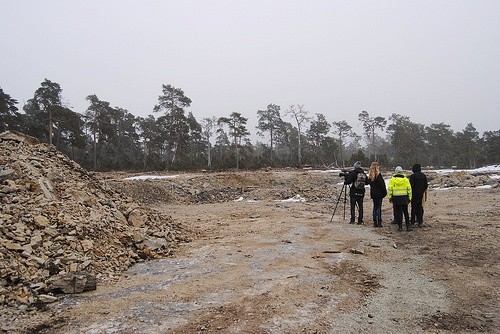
[339,169,349,177]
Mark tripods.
[330,177,359,222]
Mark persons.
[387,166,413,232]
[415,189,427,222]
[344,161,369,225]
[367,162,387,228]
[391,203,403,224]
[409,164,428,225]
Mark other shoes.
[397,228,413,231]
[373,223,382,227]
[349,220,363,225]
[410,223,423,227]
[391,221,403,224]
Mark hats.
[412,164,421,173]
[395,166,403,173]
[354,161,362,168]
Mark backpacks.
[353,170,366,189]
